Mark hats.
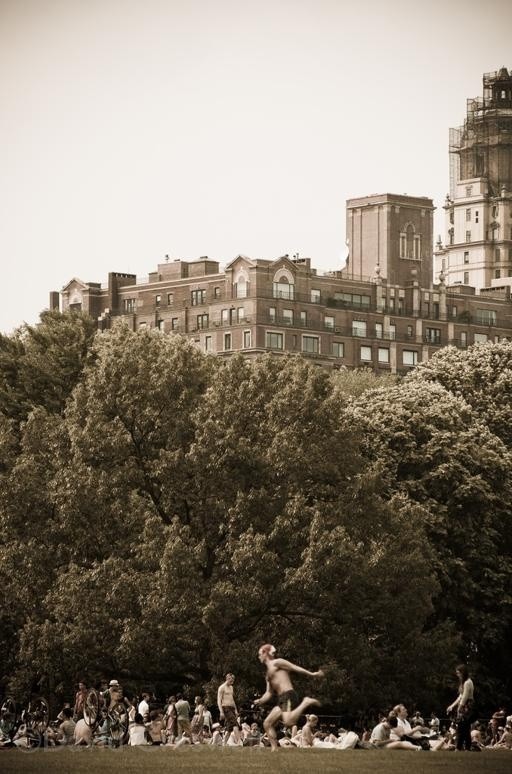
[108,679,121,686]
[259,643,277,660]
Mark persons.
[254,643,325,750]
[446,664,474,751]
[470,712,511,751]
[280,703,457,750]
[1,673,272,750]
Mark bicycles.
[83,688,129,748]
[0,696,51,747]
